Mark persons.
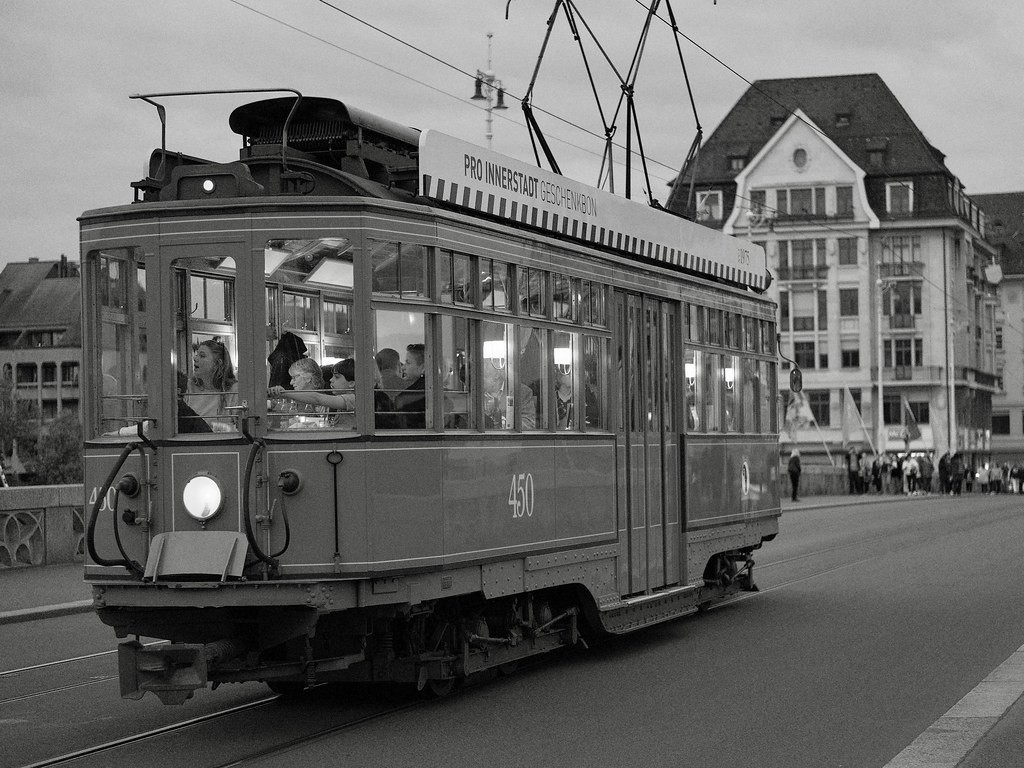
[267,356,356,430]
[845,447,864,496]
[939,451,965,497]
[185,336,237,432]
[964,464,975,492]
[373,342,599,430]
[101,371,213,437]
[268,358,330,421]
[977,462,1024,496]
[788,448,802,502]
[860,452,935,493]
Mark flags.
[784,385,815,443]
[901,393,921,441]
[841,385,866,446]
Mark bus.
[74,86,802,710]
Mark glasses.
[291,374,300,381]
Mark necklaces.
[559,390,571,396]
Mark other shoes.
[792,498,800,502]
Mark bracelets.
[118,428,120,437]
